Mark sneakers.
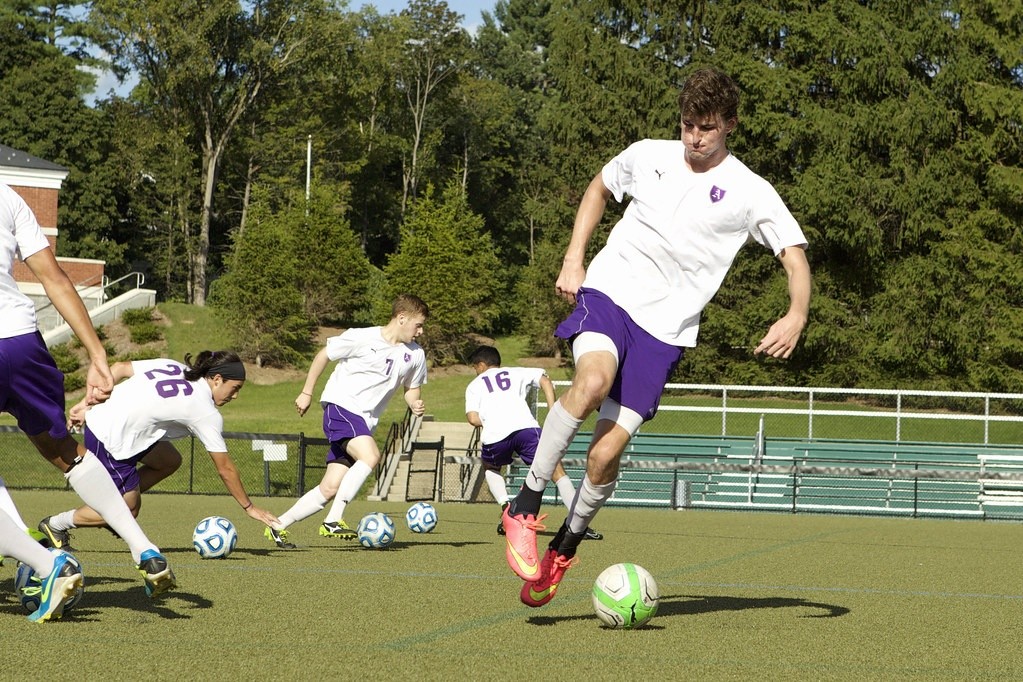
[36,515,82,554]
[519,546,581,607]
[584,528,605,541]
[134,548,179,595]
[319,520,359,540]
[499,500,547,581]
[497,521,508,536]
[19,553,84,624]
[263,524,299,546]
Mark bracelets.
[244,503,252,510]
[302,392,312,396]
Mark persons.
[466,346,604,540]
[0,181,177,624]
[38,351,282,552]
[500,73,812,608]
[264,295,430,550]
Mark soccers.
[356,512,396,549]
[14,548,84,612]
[591,563,660,629]
[406,503,437,533]
[193,516,238,559]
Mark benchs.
[507,430,1023,517]
